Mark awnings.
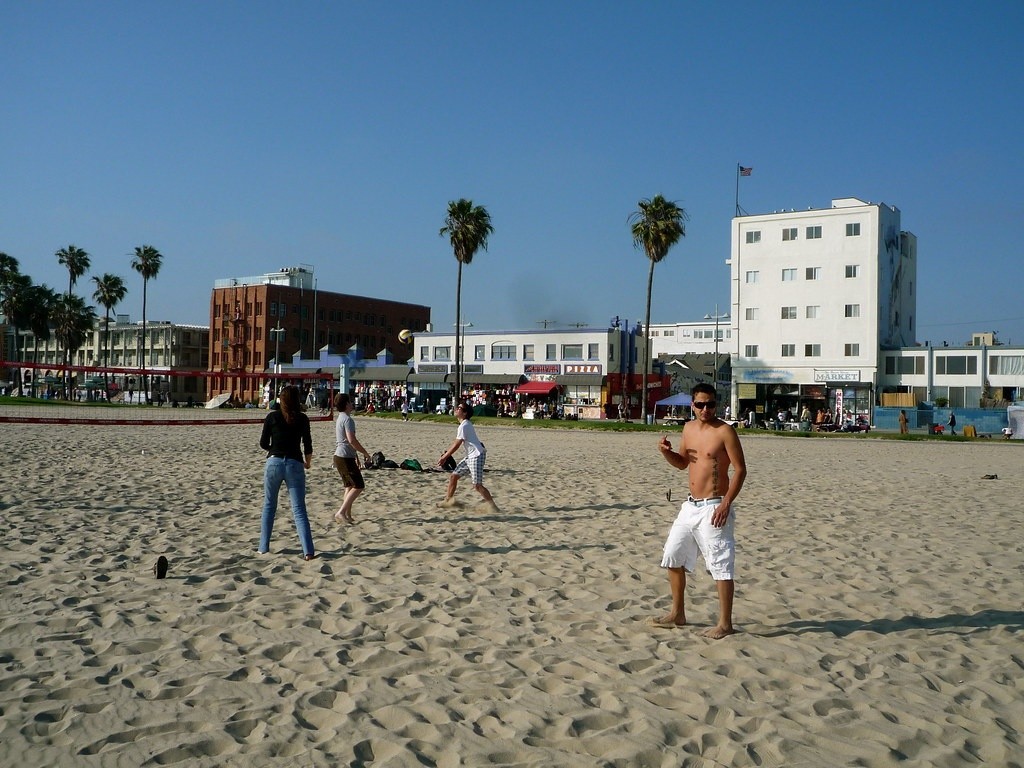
[446,373,524,384]
[516,381,556,394]
[321,367,413,380]
[407,373,449,383]
[264,368,321,374]
[556,374,608,386]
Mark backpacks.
[371,452,385,469]
[441,452,456,470]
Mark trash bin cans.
[148,399,154,406]
[802,417,810,432]
[646,414,657,425]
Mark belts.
[269,454,293,459]
[689,497,723,507]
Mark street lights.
[454,322,474,398]
[704,313,731,388]
[270,328,285,399]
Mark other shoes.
[982,474,997,479]
[153,556,169,579]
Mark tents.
[653,392,692,425]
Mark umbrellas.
[205,393,231,409]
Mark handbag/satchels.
[382,459,399,468]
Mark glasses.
[456,405,463,412]
[693,401,716,409]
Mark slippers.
[666,489,671,501]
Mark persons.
[743,407,755,429]
[219,389,316,409]
[319,391,431,422]
[158,389,193,408]
[776,405,869,433]
[333,393,370,522]
[947,412,957,436]
[899,410,908,434]
[1002,428,1013,439]
[663,406,687,426]
[438,403,501,512]
[257,386,315,560]
[459,396,564,419]
[659,384,748,639]
[1,386,134,405]
[619,401,630,419]
[723,404,731,420]
